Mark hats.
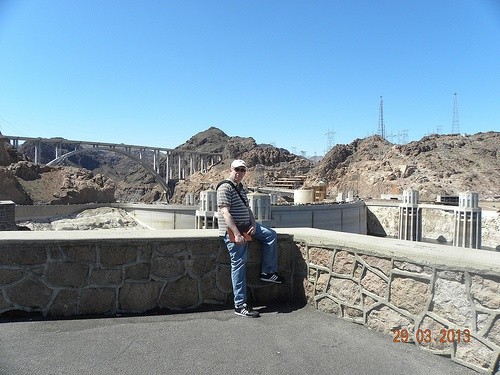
[231,160,246,169]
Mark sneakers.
[235,304,259,318]
[261,273,283,283]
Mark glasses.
[233,167,246,172]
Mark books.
[228,226,253,243]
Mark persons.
[217,159,286,318]
